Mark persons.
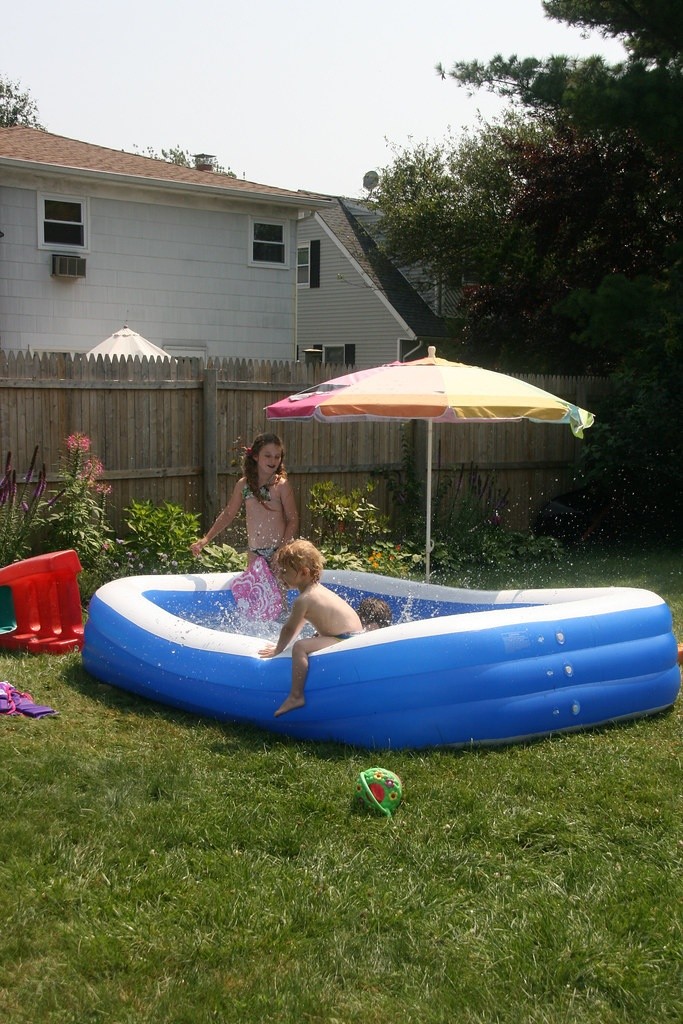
[356,598,393,632]
[190,430,298,565]
[256,539,366,718]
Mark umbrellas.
[80,325,179,364]
[263,345,597,585]
